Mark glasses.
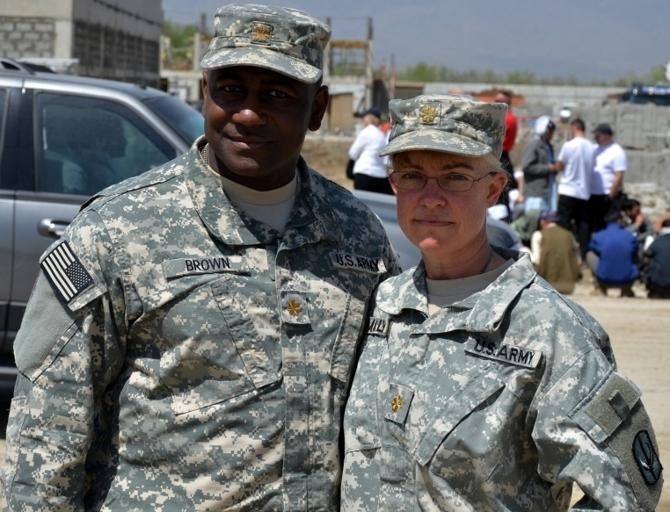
[387,157,497,194]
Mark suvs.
[0,58,526,440]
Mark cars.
[622,82,670,106]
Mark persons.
[493,92,525,225]
[586,213,638,298]
[522,114,558,220]
[529,209,584,293]
[348,108,393,195]
[620,199,667,299]
[558,118,599,259]
[337,93,665,510]
[0,2,402,511]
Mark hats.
[378,93,508,161]
[201,3,332,86]
